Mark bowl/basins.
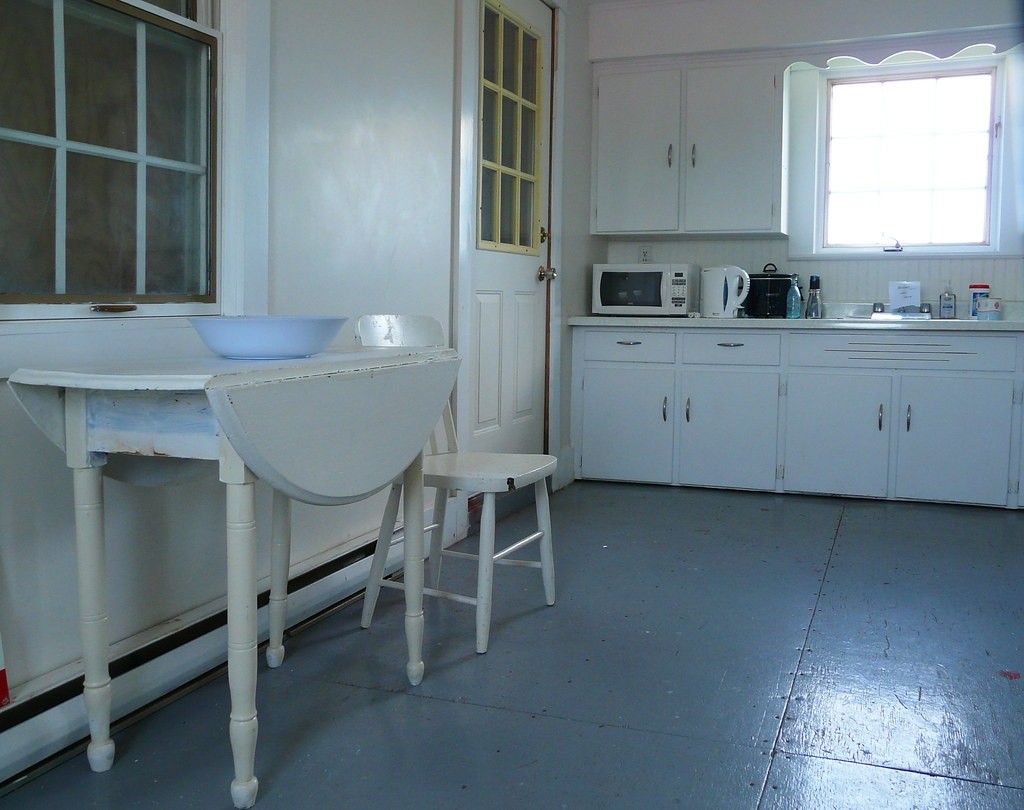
[187,313,351,360]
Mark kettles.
[700,265,750,318]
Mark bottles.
[787,272,802,319]
[968,283,990,320]
[807,275,822,319]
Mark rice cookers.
[742,262,799,318]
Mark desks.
[6,346,462,810]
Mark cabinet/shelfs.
[589,50,791,241]
[571,327,781,491]
[782,327,1024,507]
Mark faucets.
[871,303,932,320]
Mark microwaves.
[592,262,701,316]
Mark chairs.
[355,313,557,655]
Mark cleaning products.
[786,273,823,319]
[939,280,956,319]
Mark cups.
[800,300,807,319]
[977,296,1001,320]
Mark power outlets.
[639,245,652,264]
